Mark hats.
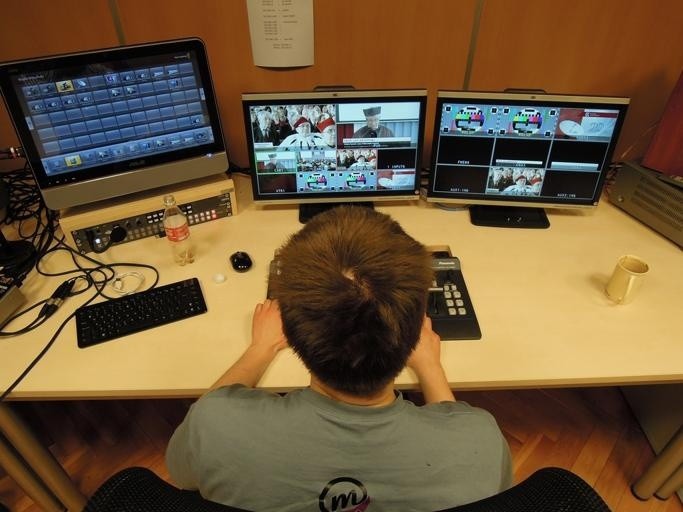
[363,107,381,116]
[294,117,309,128]
[317,118,335,133]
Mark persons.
[250,102,420,191]
[163,206,516,511]
[488,168,543,198]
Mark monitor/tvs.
[241,85,427,224]
[427,88,632,228]
[0,36,230,211]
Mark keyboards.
[75,277,207,349]
[267,256,482,341]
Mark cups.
[604,255,651,305]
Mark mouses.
[229,251,252,273]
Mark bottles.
[162,195,195,266]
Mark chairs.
[84,467,611,512]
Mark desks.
[0,178,683,511]
